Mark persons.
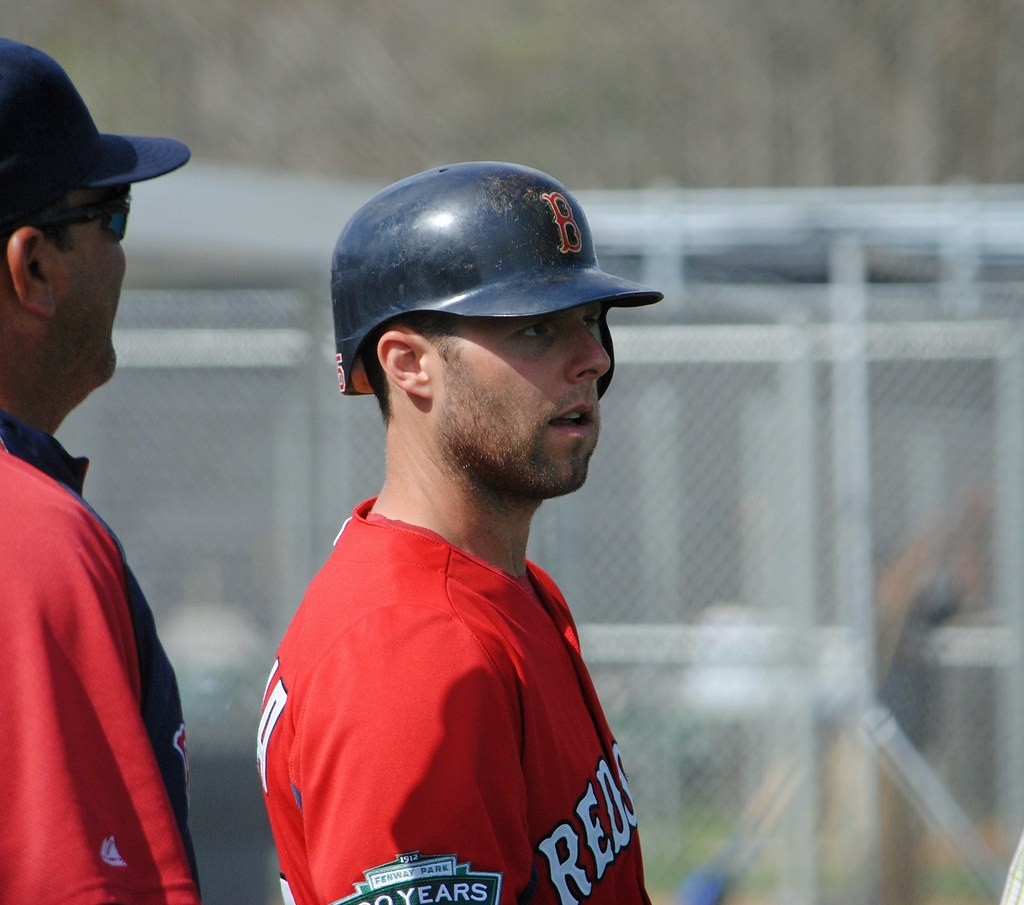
[255,161,664,905]
[0,39,201,905]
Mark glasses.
[32,185,132,242]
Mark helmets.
[329,158,664,397]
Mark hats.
[2,35,193,234]
